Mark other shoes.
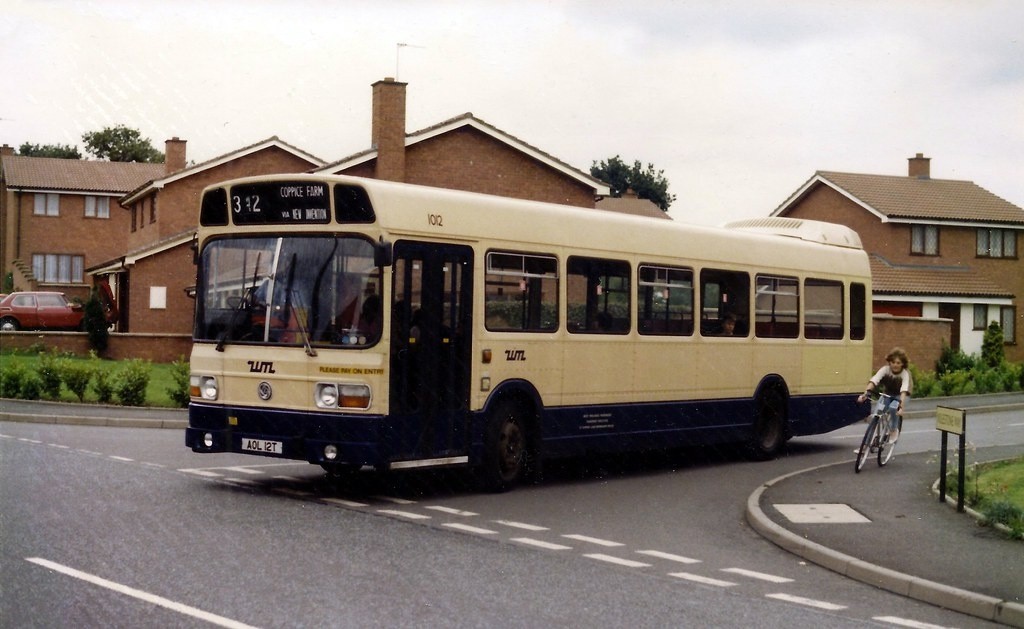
[889,428,899,444]
[853,445,866,454]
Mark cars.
[0,291,86,331]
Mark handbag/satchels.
[103,306,113,321]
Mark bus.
[181,172,874,493]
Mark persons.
[853,348,913,454]
[595,312,613,332]
[237,253,298,343]
[715,312,737,336]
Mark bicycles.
[855,390,902,475]
